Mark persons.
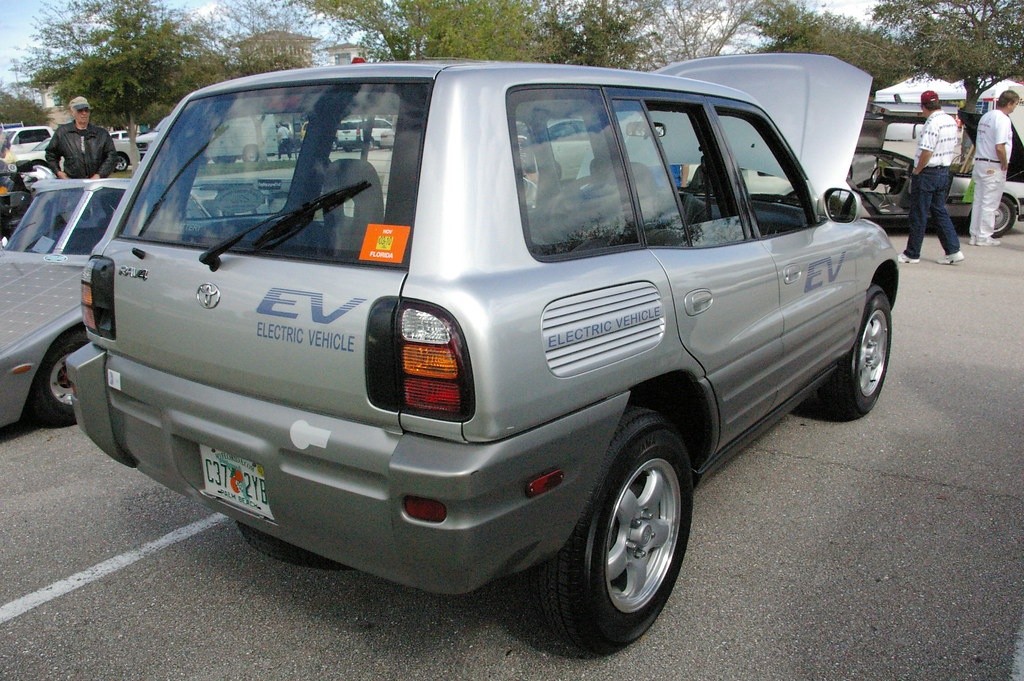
[524,162,562,193]
[969,90,1020,246]
[46,96,117,179]
[648,165,689,192]
[277,121,294,161]
[897,91,964,264]
[0,123,17,247]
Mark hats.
[69,96,90,110]
[921,91,939,104]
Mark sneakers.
[898,253,920,263]
[937,251,964,264]
[969,237,1000,246]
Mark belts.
[975,158,1001,163]
[924,166,949,169]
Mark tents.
[875,71,1024,102]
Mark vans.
[136,112,282,162]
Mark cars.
[13,132,143,173]
[108,131,139,141]
[64,52,898,657]
[0,178,204,435]
[3,125,55,160]
[299,121,309,143]
[681,119,1024,240]
[337,118,395,153]
